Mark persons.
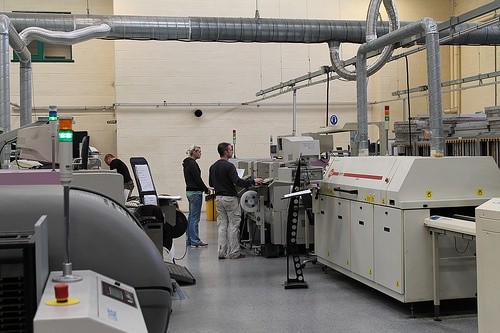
[104,154,135,202]
[182,145,211,248]
[209,142,265,259]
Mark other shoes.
[237,254,245,258]
[190,242,208,248]
[219,257,225,259]
